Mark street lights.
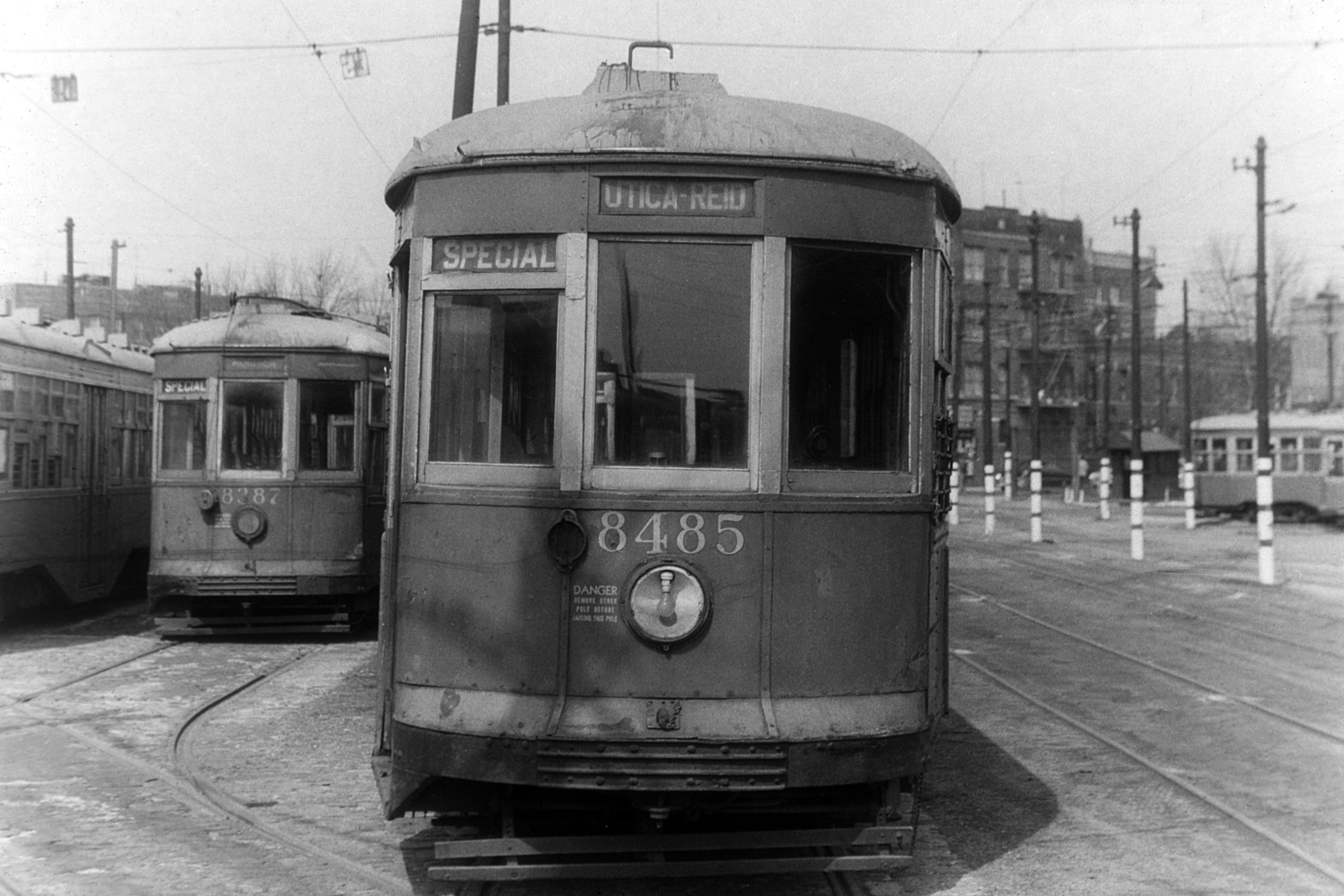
[967,208,1211,562]
[1232,133,1295,589]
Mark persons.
[1072,453,1089,502]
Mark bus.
[370,44,961,887]
[0,309,153,625]
[1189,401,1343,525]
[148,291,395,640]
